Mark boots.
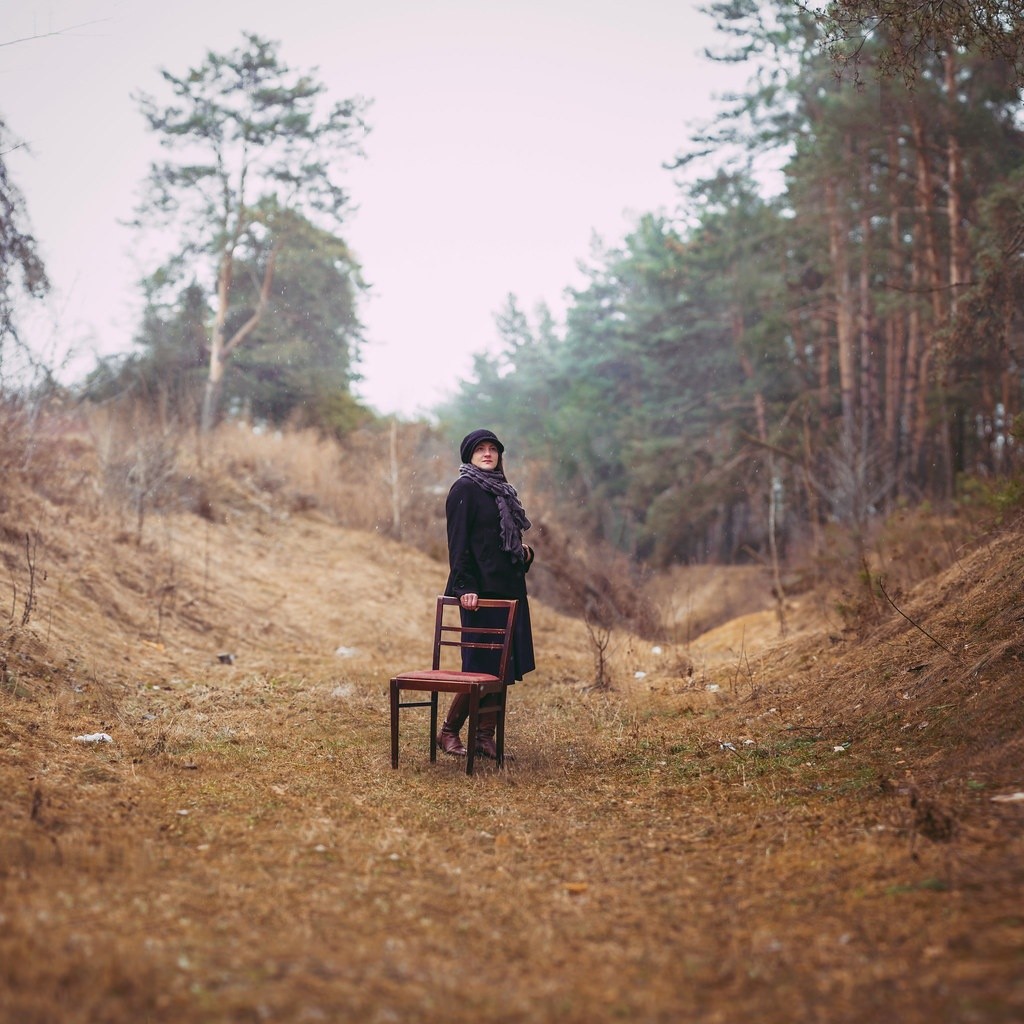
[436,693,488,757]
[476,694,498,761]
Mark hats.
[460,429,504,464]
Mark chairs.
[390,596,518,777]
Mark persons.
[434,428,537,760]
